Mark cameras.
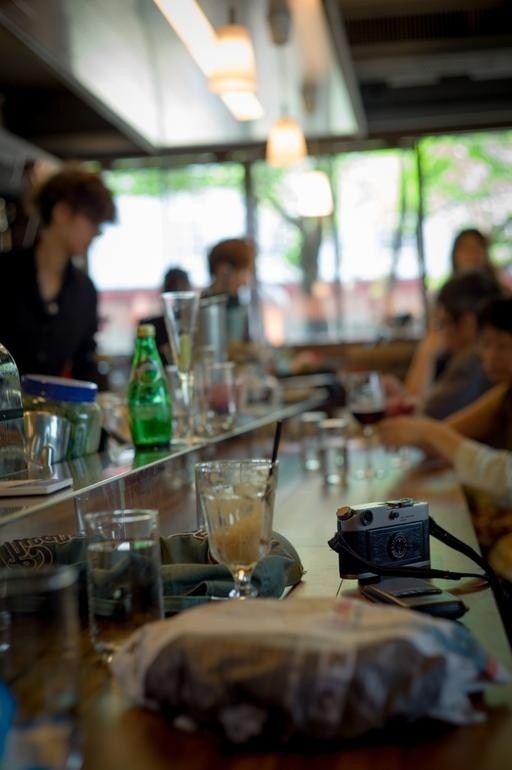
[336,497,431,586]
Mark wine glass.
[193,454,281,603]
[345,370,423,488]
[80,503,170,731]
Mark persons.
[138,237,255,365]
[378,228,511,600]
[1,168,116,390]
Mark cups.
[301,412,328,474]
[319,421,350,486]
[30,462,74,488]
[0,560,92,770]
[24,410,69,463]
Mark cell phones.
[361,577,470,620]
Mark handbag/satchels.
[0,528,304,616]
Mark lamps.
[265,0,308,167]
[294,84,333,216]
[209,1,257,94]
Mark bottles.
[1,344,27,475]
[22,375,104,463]
[156,288,283,443]
[123,323,176,453]
